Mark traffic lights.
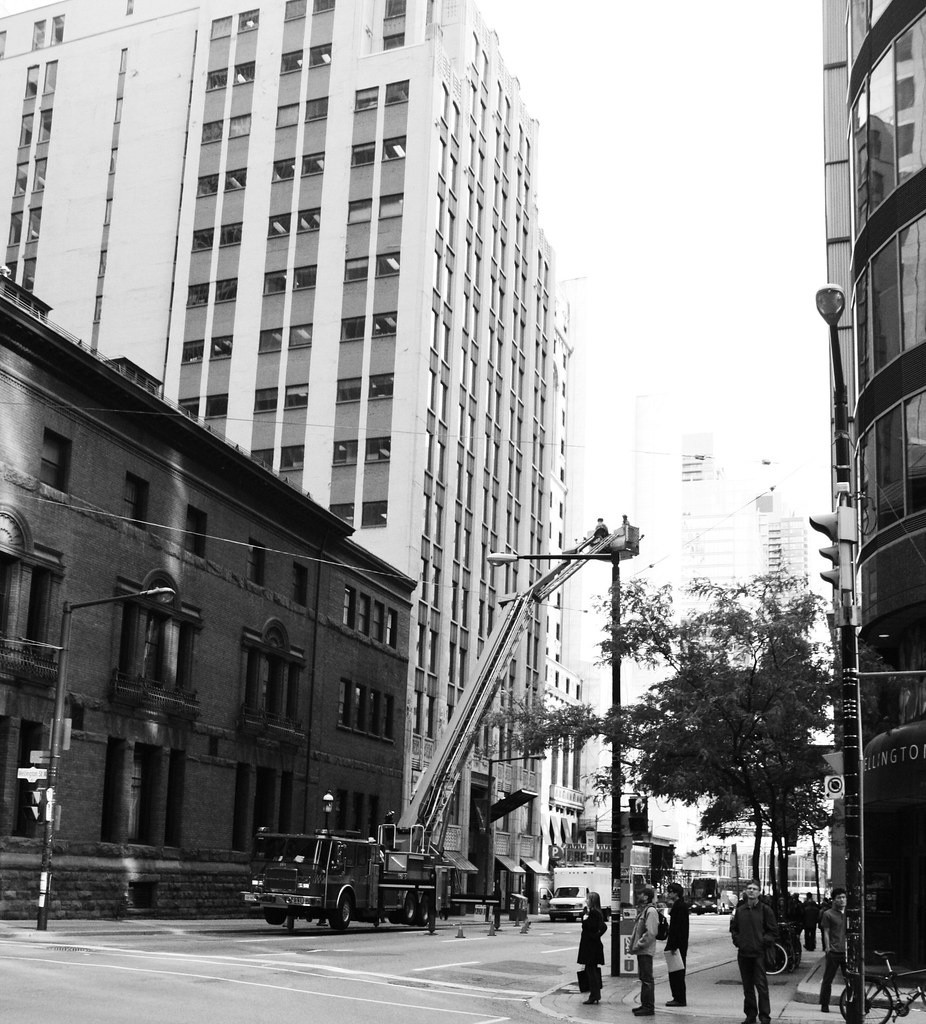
[821,751,843,778]
[26,790,41,804]
[24,806,37,824]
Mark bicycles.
[765,923,804,976]
[839,950,926,1024]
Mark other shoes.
[761,1017,771,1024]
[635,1008,653,1016]
[821,1004,829,1012]
[741,1017,756,1024]
[666,1000,686,1006]
[632,1006,642,1012]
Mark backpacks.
[645,906,668,940]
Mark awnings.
[496,855,526,874]
[443,851,479,874]
[521,857,549,875]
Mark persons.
[622,515,630,525]
[664,883,689,1006]
[596,518,609,531]
[629,889,659,1016]
[793,892,831,951]
[577,892,607,1004]
[732,880,780,1024]
[820,888,846,1012]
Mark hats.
[635,888,654,897]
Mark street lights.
[38,586,176,934]
[481,753,548,905]
[487,549,620,979]
[595,803,631,868]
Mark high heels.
[583,995,601,1005]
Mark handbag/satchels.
[577,968,602,993]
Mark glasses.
[668,890,675,893]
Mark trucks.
[546,867,614,923]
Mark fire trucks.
[238,523,646,927]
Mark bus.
[690,877,752,915]
